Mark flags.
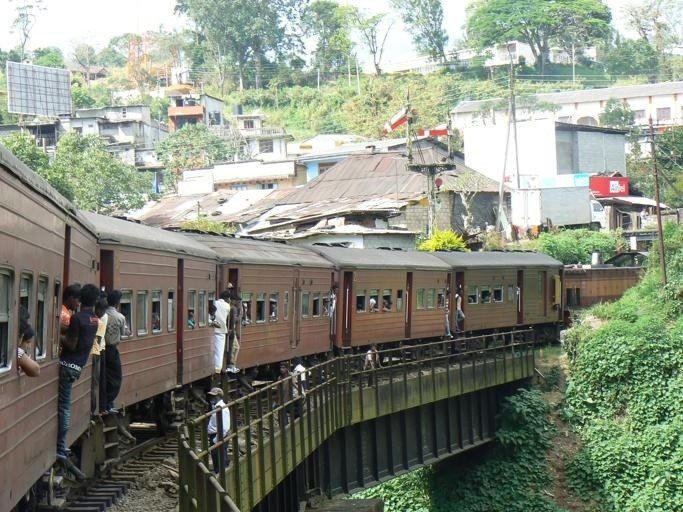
[417,120,448,139]
[382,105,410,135]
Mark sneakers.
[226,366,240,374]
[90,408,119,426]
[448,335,453,339]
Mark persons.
[274,361,298,427]
[454,289,465,331]
[291,356,306,392]
[363,343,382,388]
[19,283,131,461]
[445,290,451,335]
[204,387,231,475]
[151,288,389,375]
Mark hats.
[207,387,224,398]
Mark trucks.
[509,184,607,235]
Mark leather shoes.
[58,447,72,456]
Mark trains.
[0,143,648,510]
